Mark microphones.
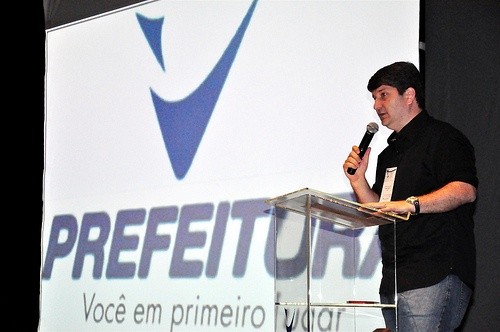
[348,123,379,175]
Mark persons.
[343,61,478,331]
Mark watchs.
[406,196,422,215]
